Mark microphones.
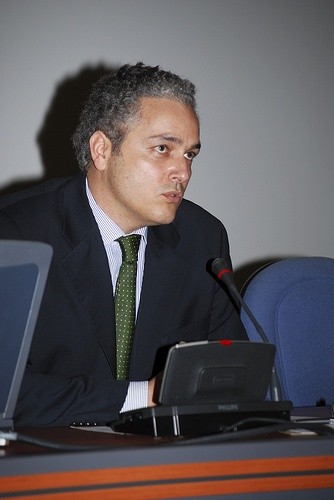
[211,257,283,401]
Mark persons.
[0,62,250,426]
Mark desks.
[0,422,334,500]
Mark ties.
[114,234,143,381]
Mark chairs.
[239,257,334,407]
